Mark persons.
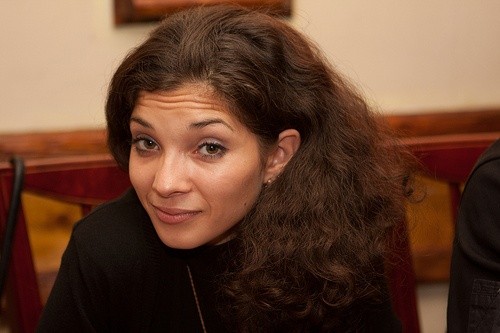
[448,139,500,332]
[33,7,422,333]
